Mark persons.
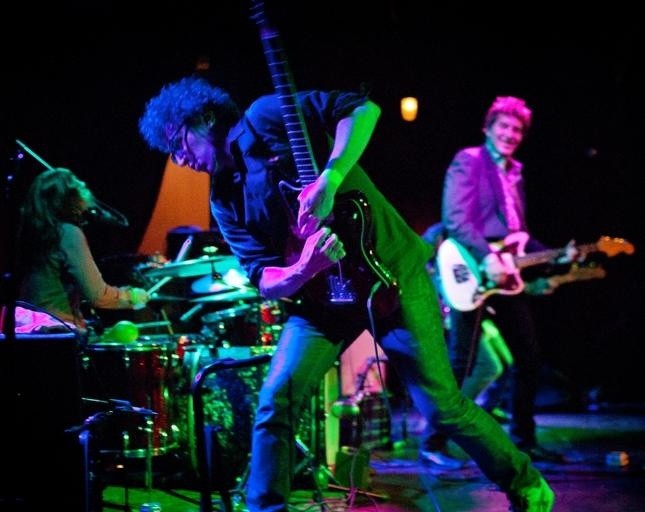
[416,94,586,474]
[140,75,558,512]
[421,217,515,425]
[22,164,151,421]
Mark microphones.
[87,209,118,223]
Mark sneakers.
[509,478,557,511]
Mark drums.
[134,332,206,417]
[188,343,340,491]
[200,300,287,347]
[76,341,187,460]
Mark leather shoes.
[420,447,466,471]
[525,445,564,464]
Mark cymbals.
[144,255,243,279]
[191,275,251,293]
[188,286,259,303]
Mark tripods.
[293,390,390,512]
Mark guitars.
[250,3,399,321]
[436,232,635,312]
[525,261,605,296]
